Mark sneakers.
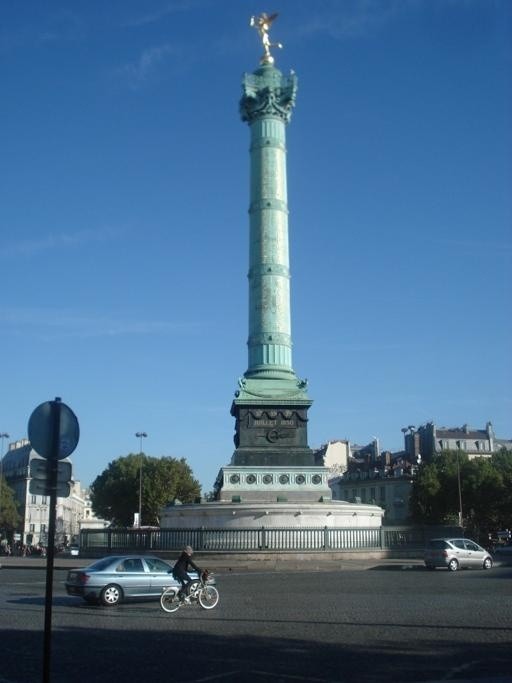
[179,589,186,601]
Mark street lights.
[133,429,149,526]
[0,432,9,462]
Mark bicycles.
[158,566,223,612]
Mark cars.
[61,546,79,557]
[422,537,495,571]
[65,555,217,607]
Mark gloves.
[201,569,205,572]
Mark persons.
[173,546,204,599]
[3,539,64,561]
